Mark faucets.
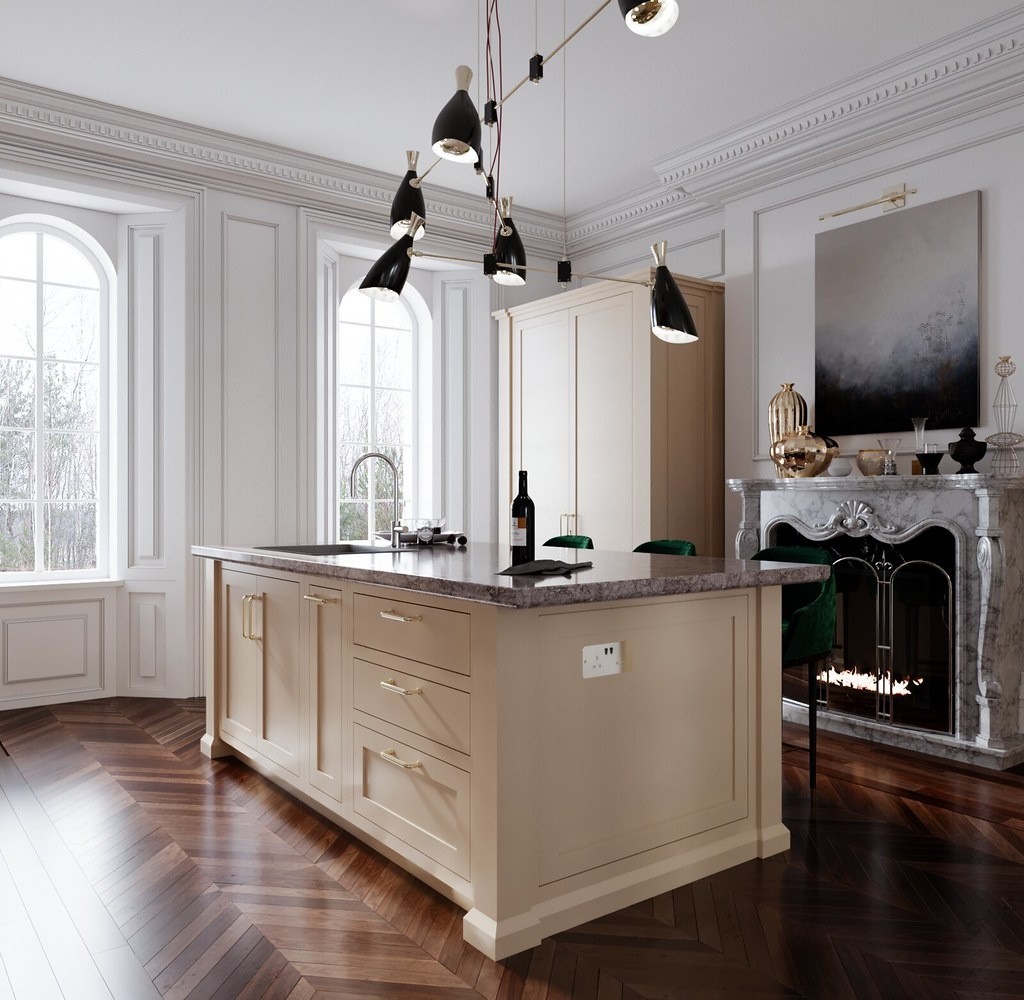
[351,453,408,548]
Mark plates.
[373,531,465,542]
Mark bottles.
[510,471,535,568]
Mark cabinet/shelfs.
[192,541,831,961]
[491,267,723,558]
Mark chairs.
[541,536,594,549]
[633,539,696,557]
[748,544,836,785]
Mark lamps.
[358,0,699,345]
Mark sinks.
[251,544,419,556]
